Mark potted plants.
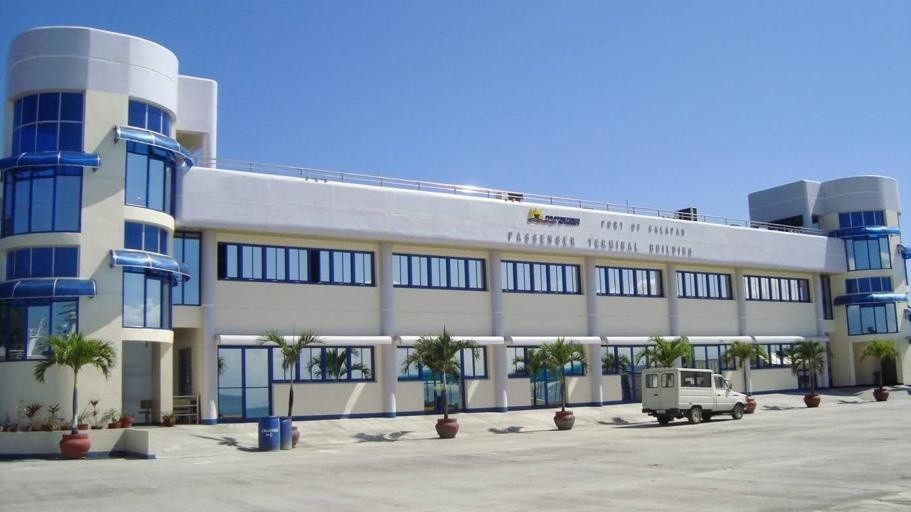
[527,338,591,429]
[1,329,137,459]
[401,320,481,438]
[719,340,769,413]
[257,321,325,448]
[859,339,904,400]
[787,340,832,407]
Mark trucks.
[641,367,748,422]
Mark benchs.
[138,393,200,424]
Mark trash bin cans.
[258,415,293,451]
